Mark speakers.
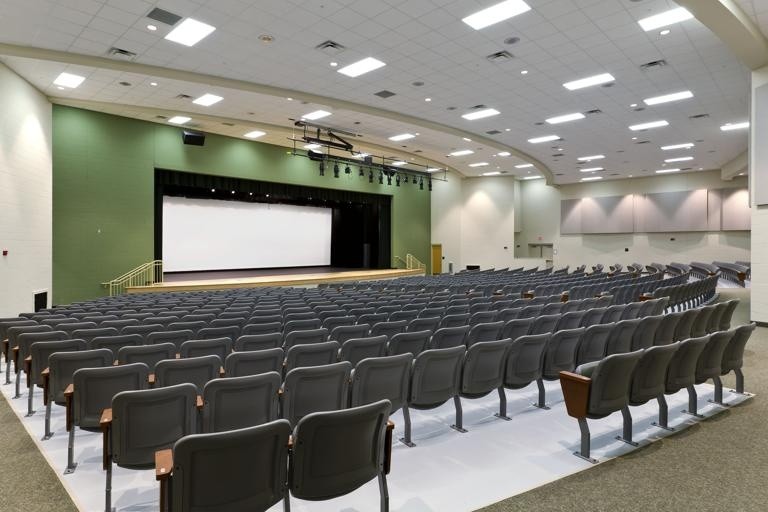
[182,131,205,145]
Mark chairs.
[104,309,136,317]
[340,335,389,393]
[23,338,89,418]
[156,310,190,319]
[653,312,683,346]
[81,315,119,326]
[223,347,285,378]
[69,306,96,311]
[469,310,499,330]
[73,285,327,304]
[71,327,120,344]
[673,308,700,341]
[155,418,291,512]
[142,316,180,328]
[283,318,322,338]
[284,328,330,350]
[179,336,234,360]
[606,317,641,357]
[371,319,410,348]
[88,308,118,313]
[280,302,307,312]
[286,341,340,374]
[192,308,222,316]
[408,344,468,433]
[501,317,536,339]
[201,304,229,310]
[430,325,471,350]
[696,329,737,407]
[152,304,178,310]
[209,317,246,331]
[349,353,416,448]
[99,304,125,309]
[121,305,149,312]
[460,337,514,422]
[56,309,86,316]
[330,322,371,348]
[318,261,751,315]
[147,354,221,390]
[120,312,155,322]
[630,340,682,431]
[0,320,39,373]
[632,314,664,351]
[283,360,352,434]
[40,317,80,329]
[196,324,241,349]
[505,331,553,410]
[120,324,165,340]
[167,320,208,335]
[543,326,585,381]
[721,321,756,396]
[252,308,281,316]
[0,316,30,322]
[439,310,471,330]
[387,329,433,356]
[54,321,97,337]
[217,310,250,321]
[254,304,280,311]
[116,341,182,367]
[56,304,80,309]
[466,319,506,351]
[140,307,170,315]
[248,315,282,324]
[406,315,442,336]
[576,322,617,370]
[321,316,357,336]
[31,314,67,323]
[98,382,204,512]
[531,312,562,336]
[288,398,395,512]
[558,348,645,465]
[283,311,317,328]
[90,333,145,359]
[99,319,139,334]
[68,312,103,320]
[282,306,313,318]
[664,333,712,419]
[63,362,150,475]
[554,310,587,333]
[39,307,67,314]
[145,328,194,354]
[224,305,253,314]
[40,348,119,441]
[233,332,285,353]
[358,312,389,330]
[3,324,53,386]
[201,370,284,433]
[19,311,51,319]
[181,313,217,325]
[11,331,69,400]
[241,321,282,336]
[172,305,200,311]
